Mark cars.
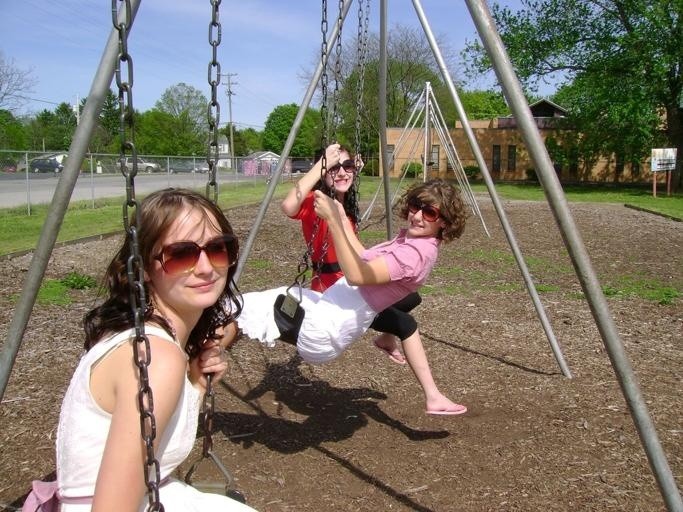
[165,159,212,174]
[0,157,16,173]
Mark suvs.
[116,157,162,174]
[31,158,64,174]
[292,161,314,173]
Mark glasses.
[326,159,355,174]
[153,235,240,275]
[407,196,446,222]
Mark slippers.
[424,403,467,416]
[373,338,406,365]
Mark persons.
[282,144,468,416]
[56,191,257,512]
[210,177,470,362]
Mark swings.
[272,1,371,344]
[110,0,246,512]
